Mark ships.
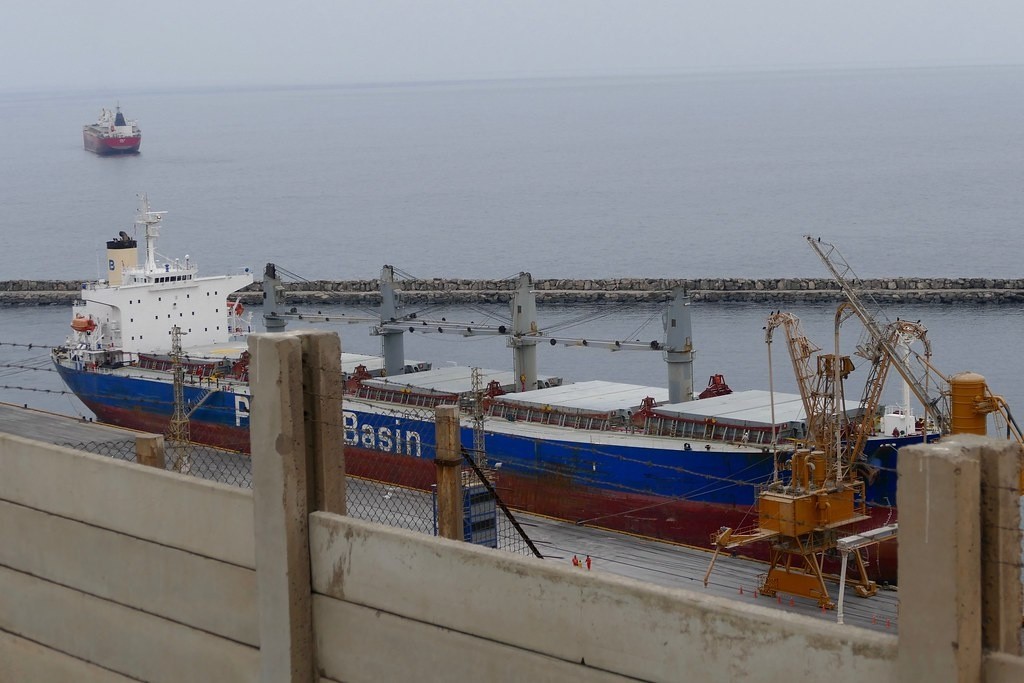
[49,188,1015,583]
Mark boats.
[227,301,244,316]
[138,351,230,377]
[70,313,98,333]
[82,100,143,156]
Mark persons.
[915,418,924,430]
